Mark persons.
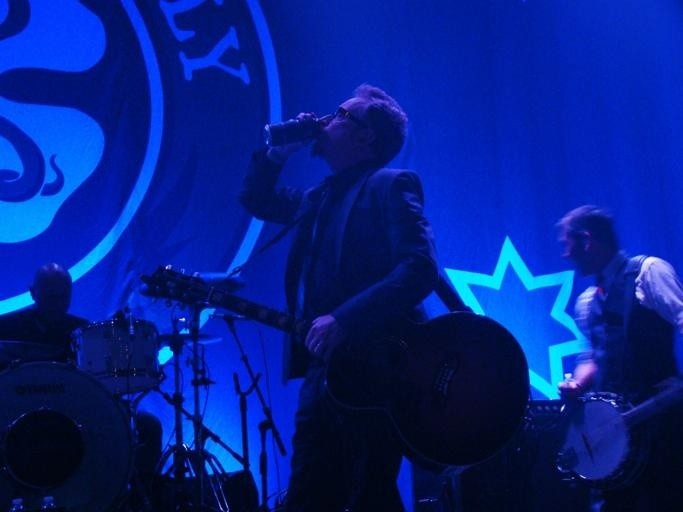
[553,201,682,511]
[0,262,163,487]
[238,81,441,512]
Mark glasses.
[332,106,365,135]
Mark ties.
[302,177,347,323]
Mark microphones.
[209,312,245,323]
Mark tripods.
[156,306,233,511]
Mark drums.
[69,315,164,395]
[0,357,139,511]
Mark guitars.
[138,266,529,468]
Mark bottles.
[38,494,56,512]
[264,113,324,147]
[7,497,26,511]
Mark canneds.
[263,116,320,146]
